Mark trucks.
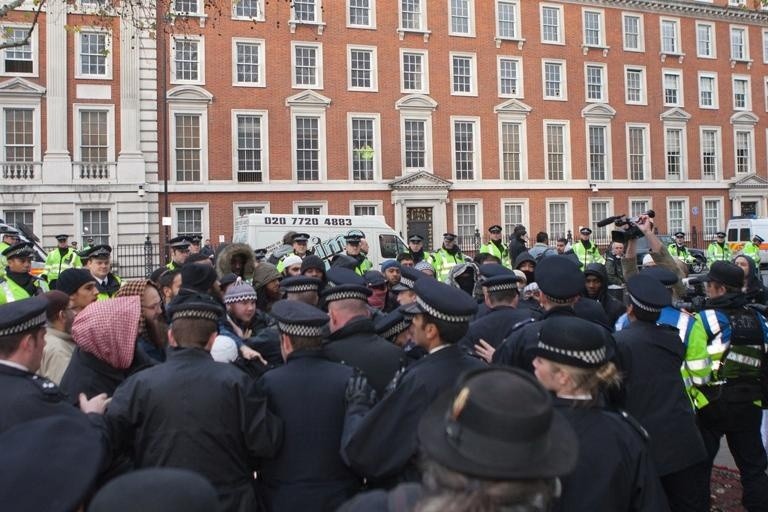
[231,211,412,271]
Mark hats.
[291,233,310,245]
[675,232,684,238]
[625,267,678,314]
[443,233,457,242]
[1,269,97,337]
[755,235,764,242]
[72,242,77,245]
[2,242,38,259]
[88,465,221,512]
[716,232,726,238]
[165,254,614,369]
[580,228,591,235]
[78,244,111,260]
[697,260,743,287]
[4,229,20,240]
[0,412,103,512]
[87,239,94,242]
[409,234,423,244]
[418,364,580,481]
[344,235,363,245]
[488,226,502,234]
[170,235,202,249]
[55,235,69,241]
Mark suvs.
[635,235,707,273]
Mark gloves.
[344,375,377,407]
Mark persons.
[0,226,768,512]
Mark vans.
[726,218,768,267]
[0,232,50,282]
